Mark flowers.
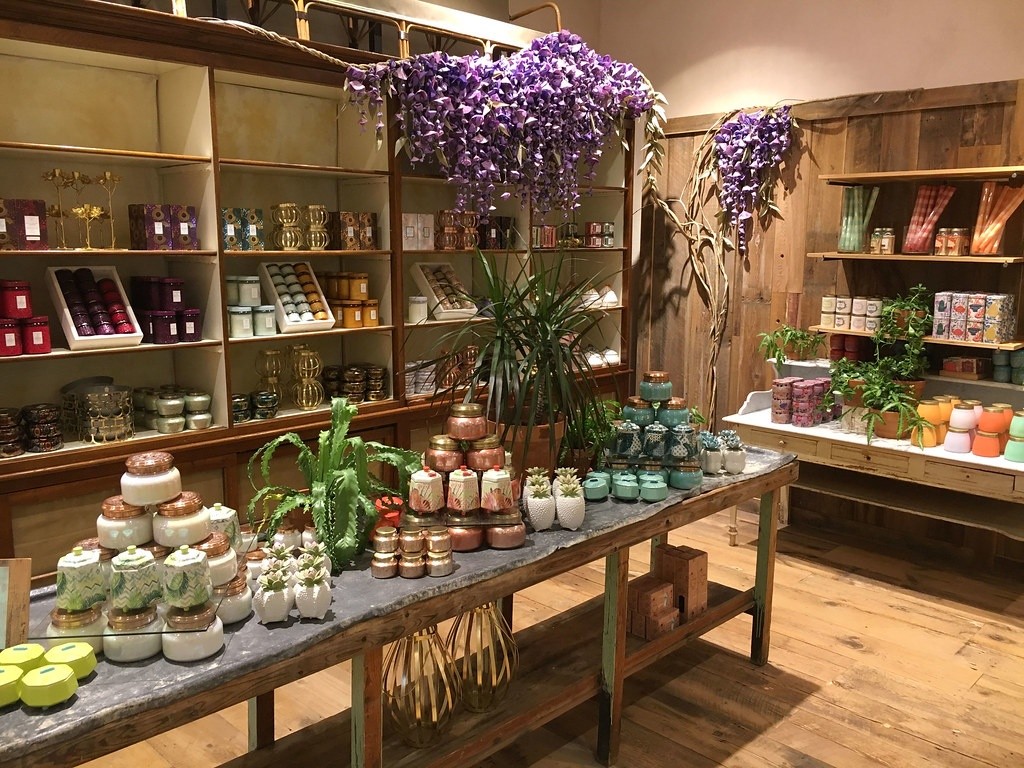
[715,103,801,261]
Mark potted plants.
[863,381,920,441]
[696,430,714,452]
[555,483,586,531]
[552,465,580,499]
[525,466,551,487]
[523,474,553,522]
[755,322,834,381]
[879,338,926,408]
[818,353,876,407]
[526,482,556,533]
[718,429,736,451]
[554,474,584,498]
[699,436,722,474]
[235,396,422,578]
[720,434,747,475]
[870,283,934,338]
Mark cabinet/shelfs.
[719,384,1024,548]
[800,165,1024,379]
[0,0,651,588]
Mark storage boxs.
[624,538,707,645]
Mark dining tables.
[2,426,806,765]
